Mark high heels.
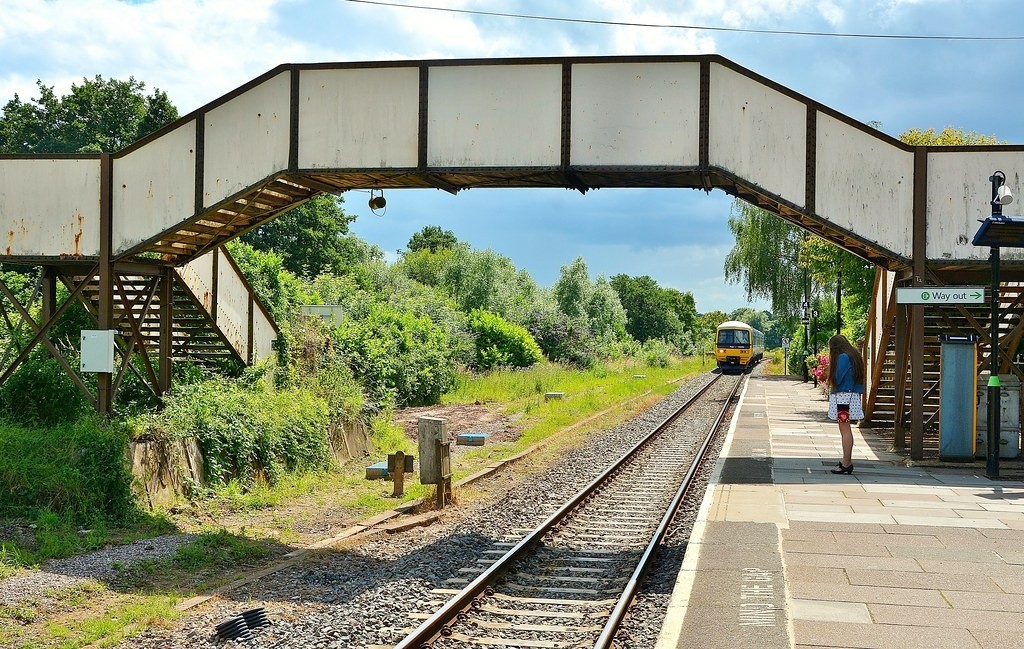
[830,461,853,474]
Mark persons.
[829,334,864,475]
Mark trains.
[714,320,764,374]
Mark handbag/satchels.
[837,403,850,424]
